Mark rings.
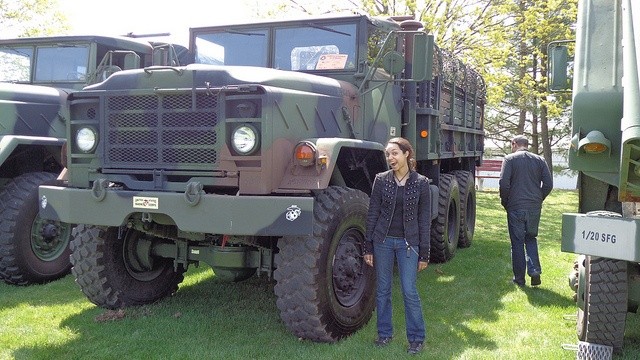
[366,260,369,264]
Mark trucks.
[0,31,189,287]
[546,0,640,352]
[38,14,488,344]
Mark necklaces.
[394,171,409,182]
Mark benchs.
[474,160,502,178]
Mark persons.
[498,135,553,287]
[362,137,431,356]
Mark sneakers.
[513,277,525,287]
[531,270,541,286]
[407,341,423,356]
[376,336,392,349]
